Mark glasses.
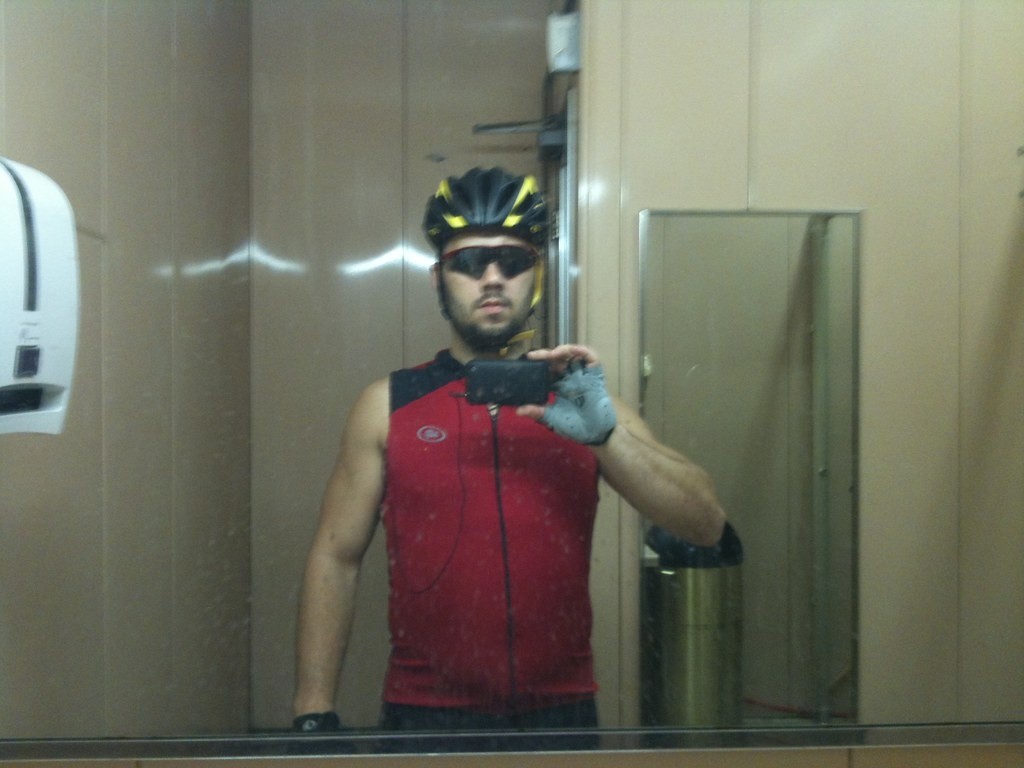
[439,245,539,280]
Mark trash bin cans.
[641,521,745,726]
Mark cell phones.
[465,357,550,406]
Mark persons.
[292,165,726,738]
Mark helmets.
[422,167,550,255]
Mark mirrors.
[0,0,1024,761]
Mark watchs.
[293,711,339,733]
